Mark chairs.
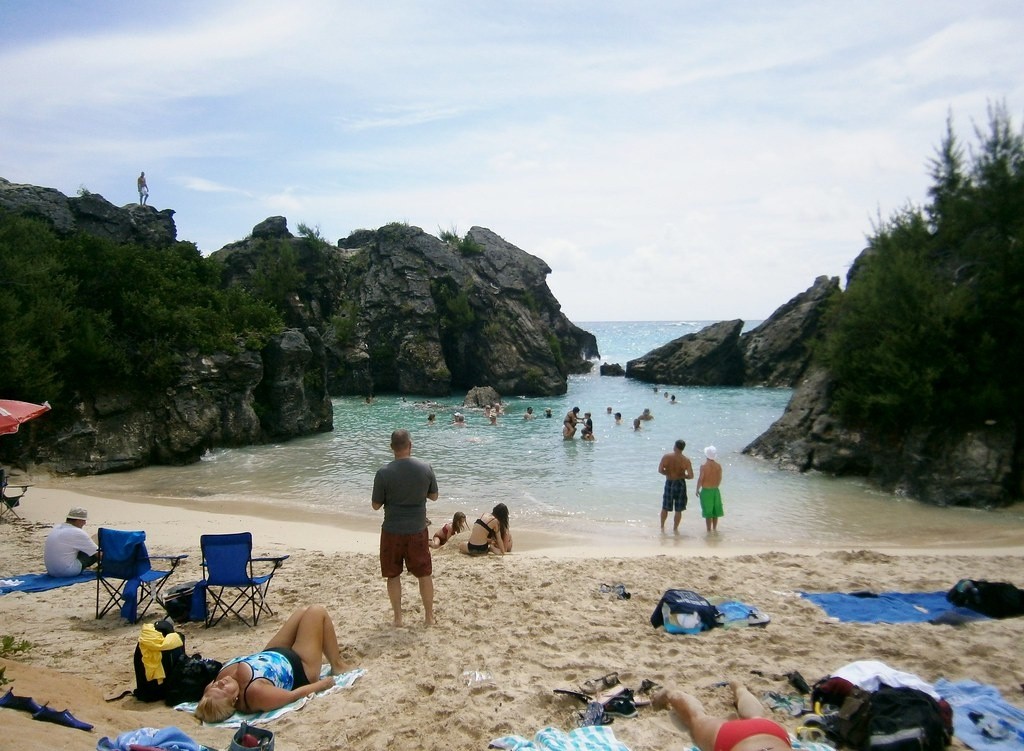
[0,467,29,521]
[95,526,190,625]
[189,531,292,628]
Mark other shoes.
[0,687,56,714]
[32,700,94,730]
[603,688,638,719]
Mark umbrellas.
[0,399,52,435]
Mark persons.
[138,172,149,205]
[562,406,595,440]
[614,412,623,425]
[372,429,438,627]
[652,679,792,751]
[658,439,694,535]
[451,412,467,425]
[195,605,359,725]
[542,407,553,417]
[427,413,436,425]
[695,446,725,531]
[44,507,102,577]
[607,407,612,414]
[484,403,503,424]
[429,512,471,548]
[654,387,678,405]
[630,419,642,429]
[524,407,534,419]
[638,408,654,420]
[459,503,513,557]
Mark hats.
[454,412,460,416]
[643,408,650,415]
[705,446,716,460]
[489,413,496,418]
[66,508,87,520]
[545,408,551,412]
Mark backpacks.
[135,621,185,703]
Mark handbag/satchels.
[232,722,274,751]
